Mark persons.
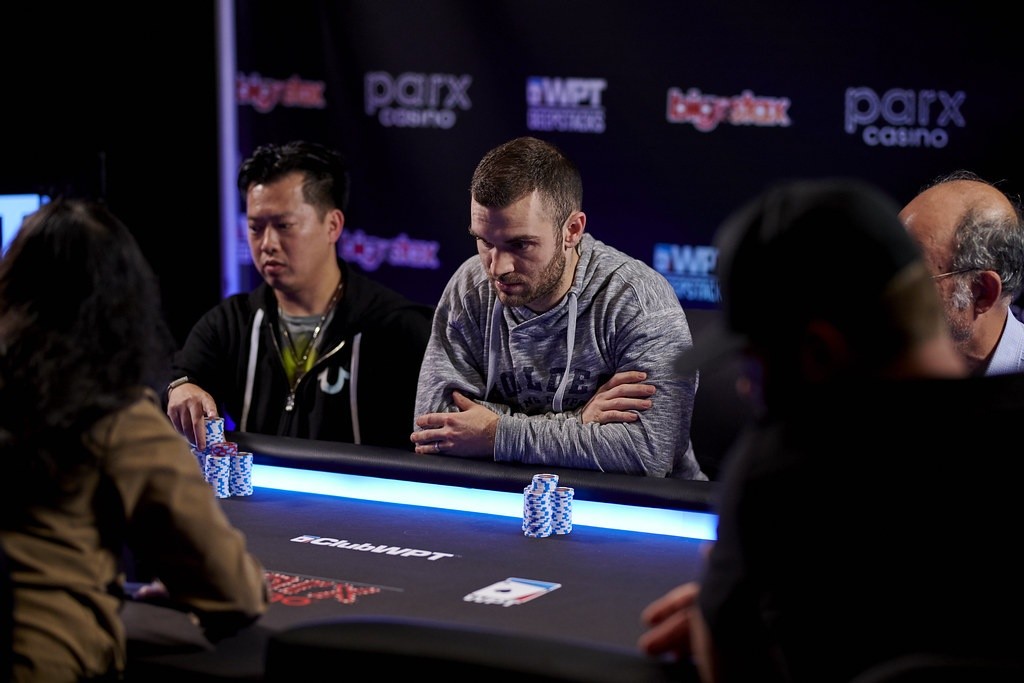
[639,178,1024,683]
[410,136,708,482]
[0,193,271,683]
[166,142,434,451]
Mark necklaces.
[280,283,344,411]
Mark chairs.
[261,616,699,683]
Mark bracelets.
[166,376,188,391]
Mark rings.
[434,441,441,454]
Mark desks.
[120,431,724,683]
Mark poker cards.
[461,576,559,609]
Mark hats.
[677,181,920,376]
